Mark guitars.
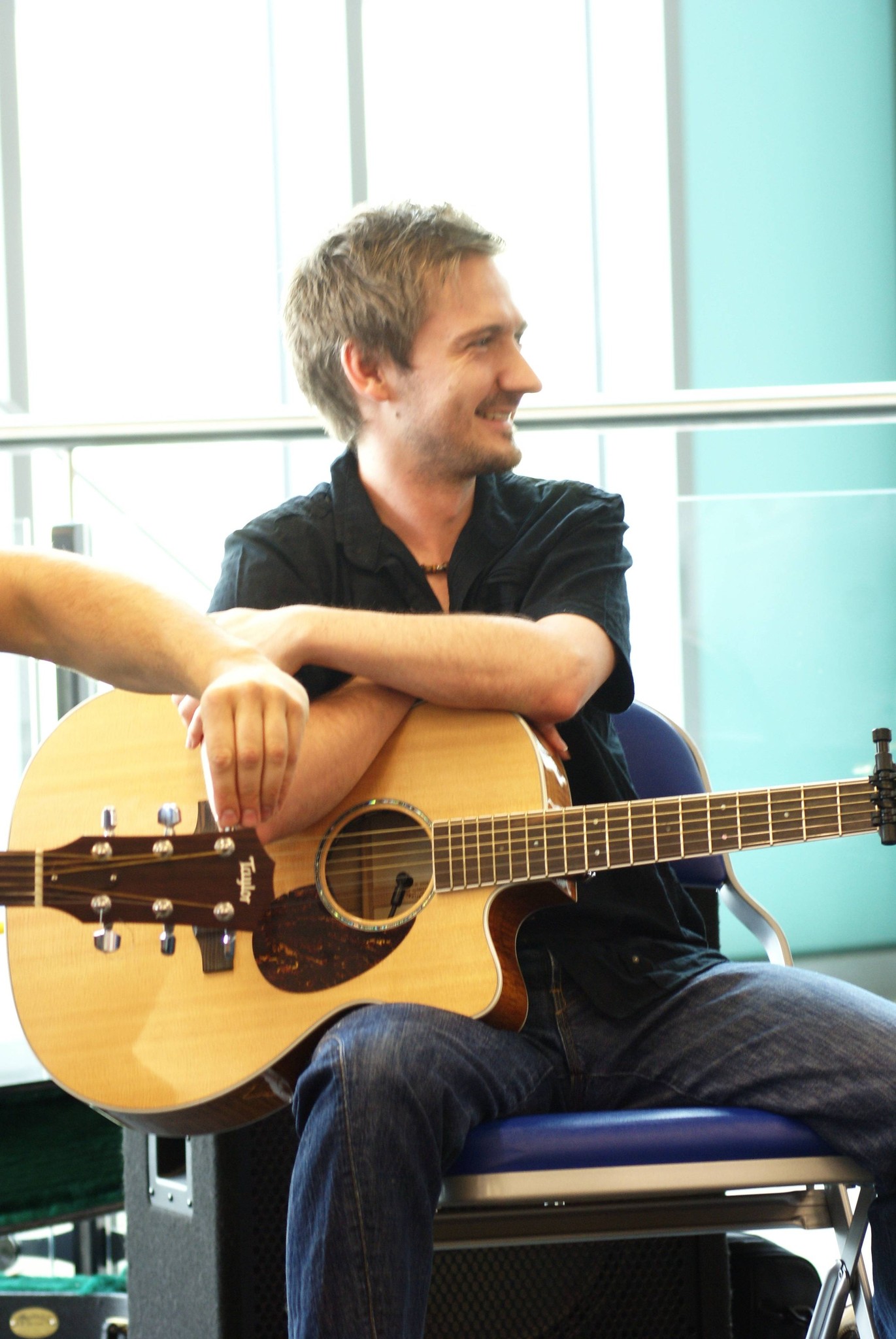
[0,676,893,1148]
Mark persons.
[2,549,309,832]
[172,198,896,1338]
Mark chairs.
[433,699,883,1338]
